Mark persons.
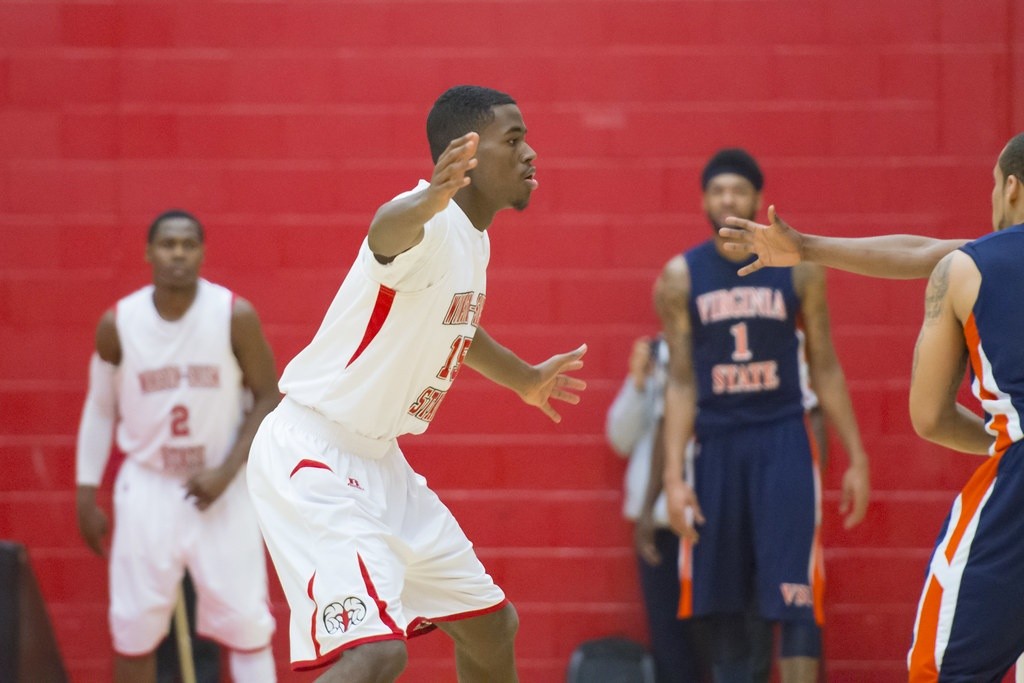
[650,146,871,683]
[246,83,588,683]
[605,277,713,682]
[719,202,979,279]
[906,131,1024,683]
[76,207,282,683]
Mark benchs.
[0,0,1024,683]
